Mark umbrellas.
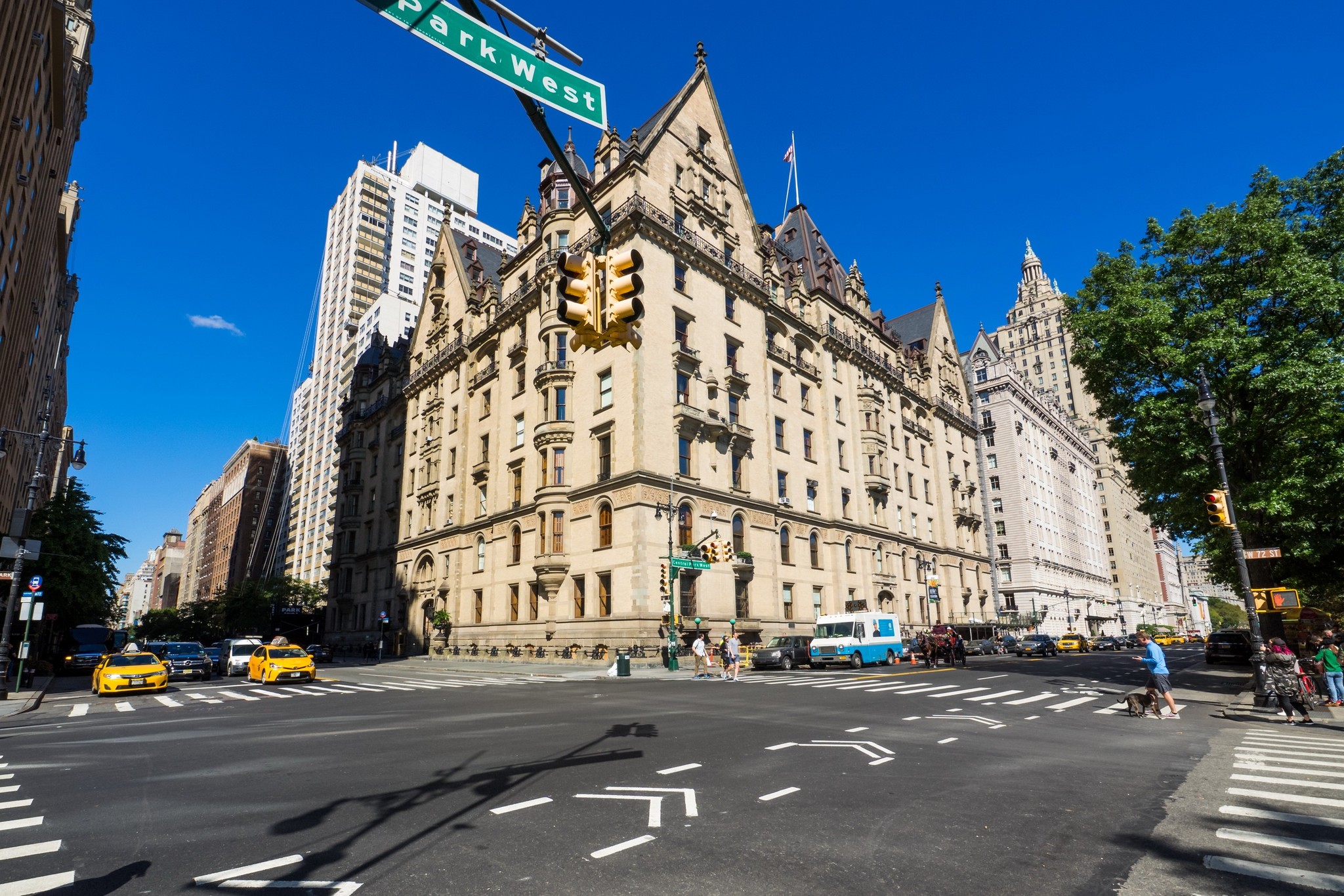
[1286,606,1331,621]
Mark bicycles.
[1296,657,1324,711]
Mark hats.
[936,620,941,623]
[1320,638,1335,645]
[1272,638,1286,650]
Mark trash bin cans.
[616,653,630,676]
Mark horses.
[916,631,938,668]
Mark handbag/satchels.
[1314,659,1324,674]
[706,657,711,667]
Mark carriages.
[915,630,966,669]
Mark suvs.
[60,623,333,699]
[751,636,815,671]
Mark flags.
[783,145,792,162]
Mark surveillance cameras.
[711,512,718,520]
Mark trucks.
[810,610,904,669]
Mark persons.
[5,643,17,683]
[1258,630,1344,725]
[723,631,741,682]
[994,634,1003,654]
[908,634,912,638]
[1135,631,1179,718]
[933,620,946,636]
[692,633,710,679]
[720,636,732,678]
[872,619,880,637]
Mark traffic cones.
[910,652,918,665]
[895,657,900,663]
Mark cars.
[964,632,1209,658]
[900,638,925,661]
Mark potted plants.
[432,608,452,637]
[682,544,698,556]
[737,552,751,563]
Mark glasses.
[726,638,729,640]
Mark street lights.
[654,494,685,670]
[1194,361,1281,708]
[0,368,89,700]
[918,554,931,630]
[1062,584,1158,636]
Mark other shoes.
[724,672,727,680]
[733,678,740,681]
[1337,700,1341,705]
[1326,699,1332,703]
[728,675,733,679]
[1324,701,1339,706]
[720,671,724,678]
[1340,702,1344,706]
[1277,708,1295,715]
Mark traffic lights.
[661,616,670,625]
[710,541,721,562]
[1203,491,1230,527]
[721,540,733,562]
[660,563,668,593]
[1264,589,1302,610]
[700,544,712,563]
[555,249,646,355]
[674,615,683,624]
[1192,599,1197,606]
[1102,599,1107,607]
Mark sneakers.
[694,676,701,679]
[703,674,710,678]
[1144,709,1162,715]
[1298,719,1314,725]
[1281,719,1296,725]
[1163,711,1180,719]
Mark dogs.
[1117,691,1161,719]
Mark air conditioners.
[32,307,40,314]
[61,299,67,308]
[780,497,790,504]
[446,519,452,524]
[424,525,430,531]
[425,436,433,442]
[10,115,24,132]
[17,174,31,187]
[32,298,37,305]
[44,468,48,474]
